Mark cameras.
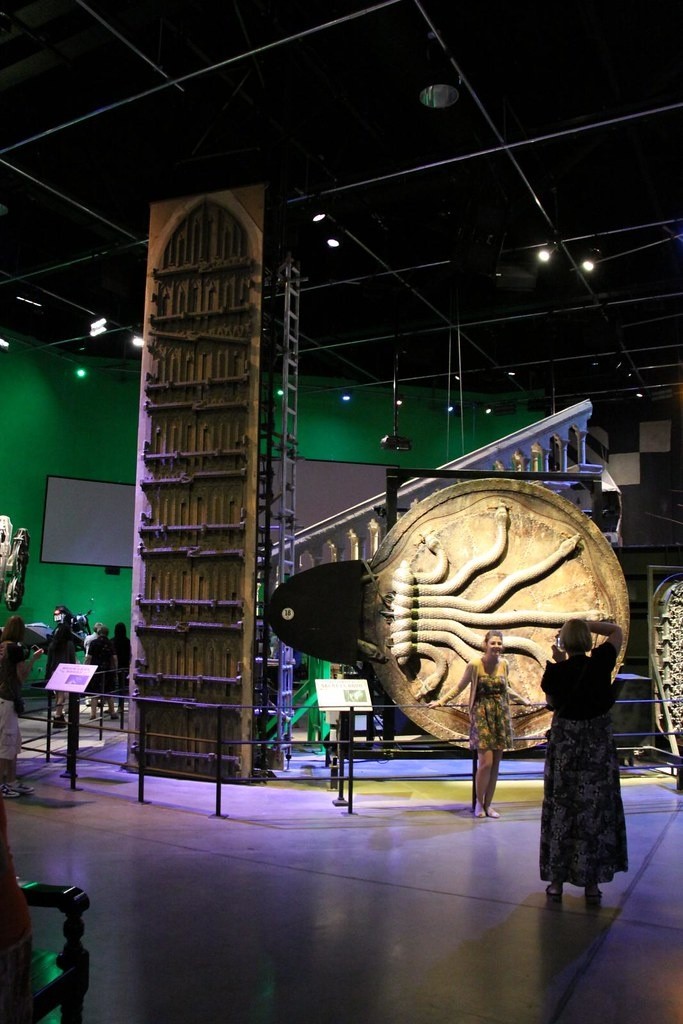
[556,636,564,648]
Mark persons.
[541,619,631,906]
[0,616,45,799]
[47,605,132,728]
[426,631,514,818]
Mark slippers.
[546,885,563,902]
[584,888,602,904]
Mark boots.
[53,714,67,728]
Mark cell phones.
[32,645,39,651]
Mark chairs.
[18,882,93,1024]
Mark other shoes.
[111,713,119,719]
[487,806,500,818]
[475,803,486,818]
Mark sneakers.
[5,780,34,795]
[0,784,21,798]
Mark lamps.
[75,194,645,451]
